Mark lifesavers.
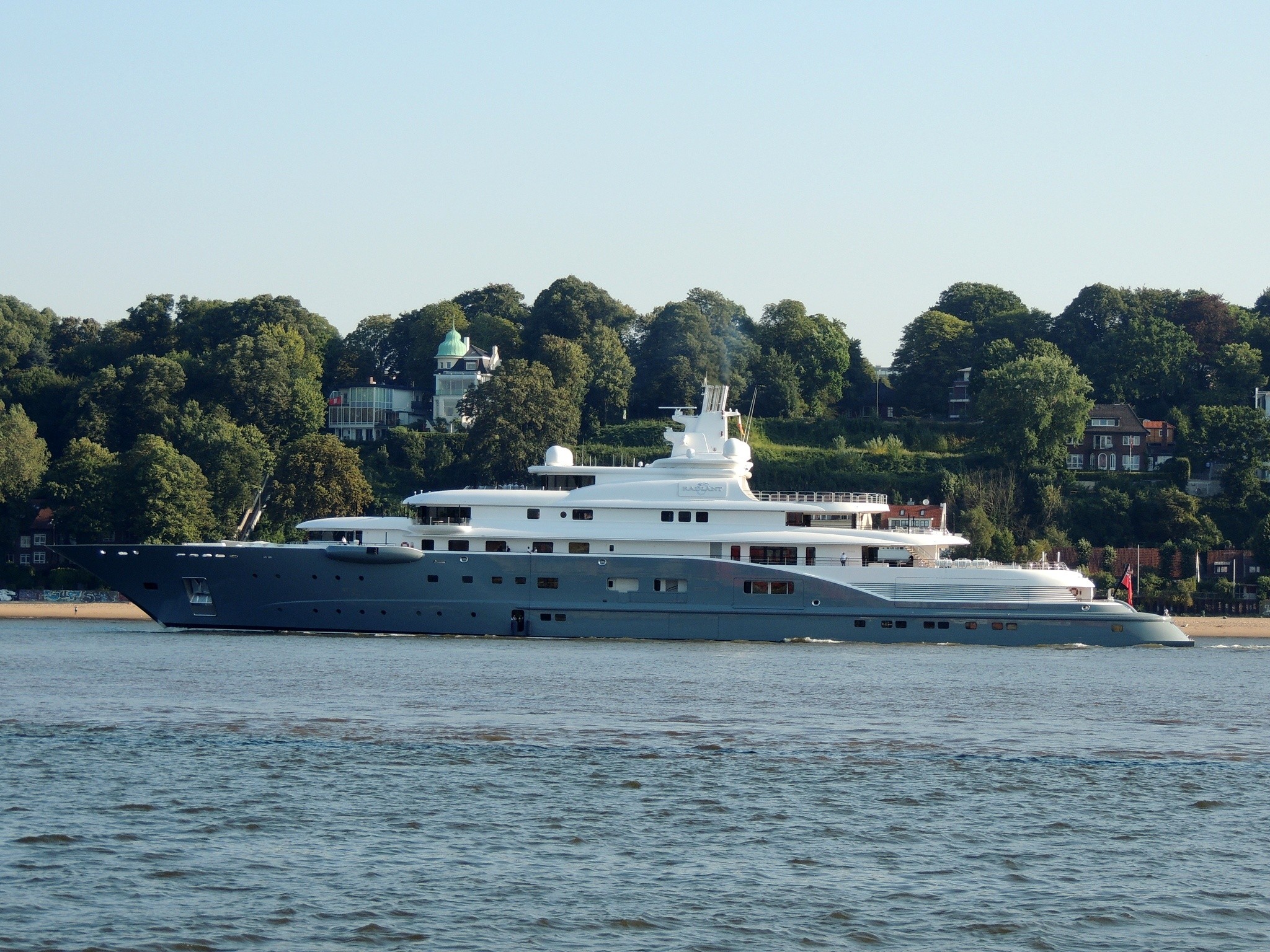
[1070,588,1079,596]
[400,541,409,547]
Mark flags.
[738,415,744,435]
[1131,439,1133,448]
[1120,565,1132,606]
[1195,553,1200,583]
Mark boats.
[42,369,1193,650]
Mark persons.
[840,552,848,566]
[527,546,537,553]
[497,545,502,552]
[514,482,518,489]
[423,517,426,524]
[507,545,510,552]
[906,556,913,567]
[504,484,507,489]
[1163,608,1168,616]
[520,483,524,489]
[507,482,513,489]
[342,536,347,545]
[75,605,78,615]
